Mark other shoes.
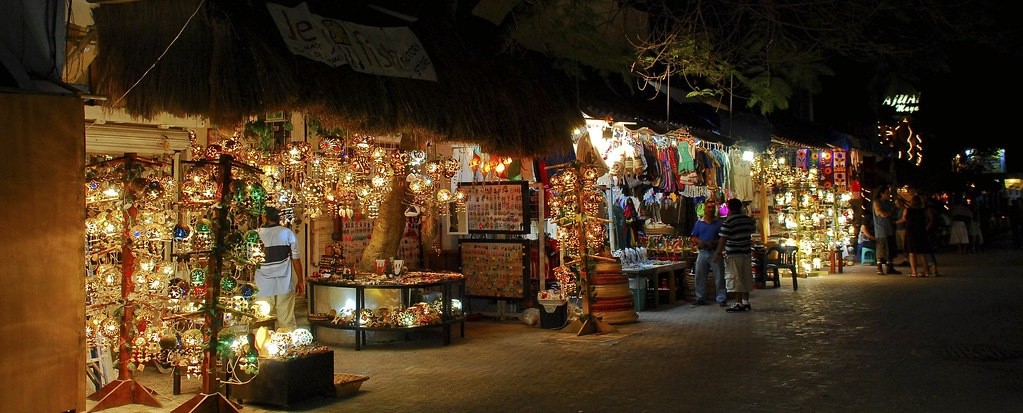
[877,269,885,275]
[887,269,902,276]
[719,301,727,307]
[691,300,707,307]
[908,272,942,277]
[726,302,751,313]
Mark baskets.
[334,373,370,398]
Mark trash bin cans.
[537,296,567,330]
[628,277,647,313]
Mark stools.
[857,244,865,261]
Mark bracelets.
[714,252,720,256]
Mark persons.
[690,201,728,308]
[247,206,303,333]
[982,197,1023,248]
[937,199,984,257]
[858,185,940,277]
[712,197,757,312]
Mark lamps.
[84,86,859,379]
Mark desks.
[226,349,336,408]
[813,249,845,274]
[622,260,687,313]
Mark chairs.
[861,248,877,265]
[764,245,799,290]
